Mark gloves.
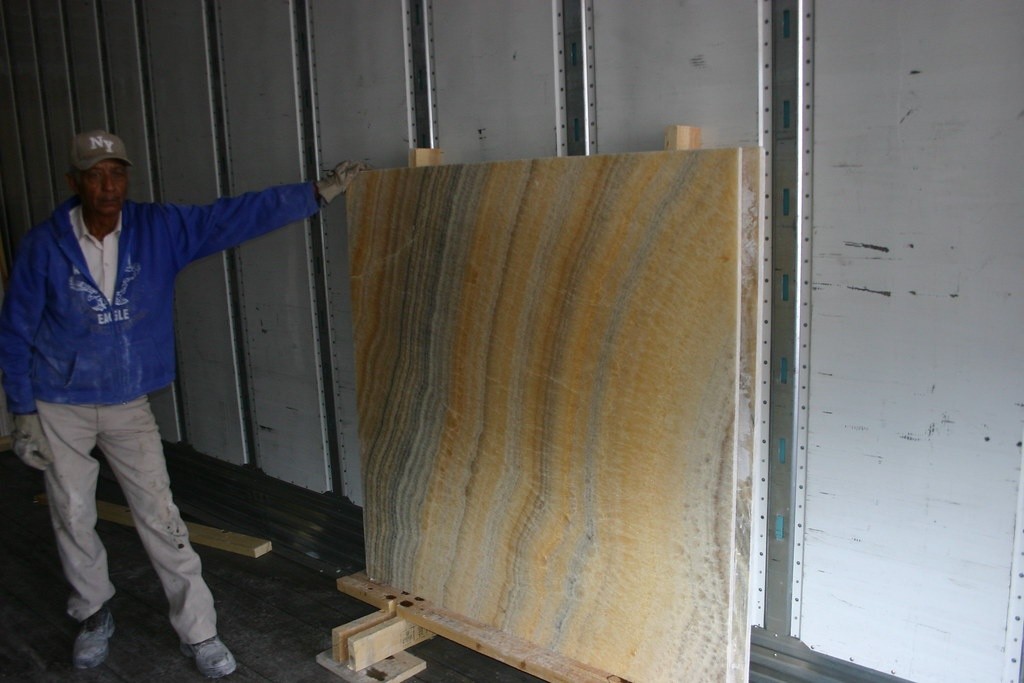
[10,414,55,471]
[316,159,374,203]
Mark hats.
[68,129,133,171]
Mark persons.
[0,130,364,677]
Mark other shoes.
[179,631,236,679]
[72,603,115,669]
